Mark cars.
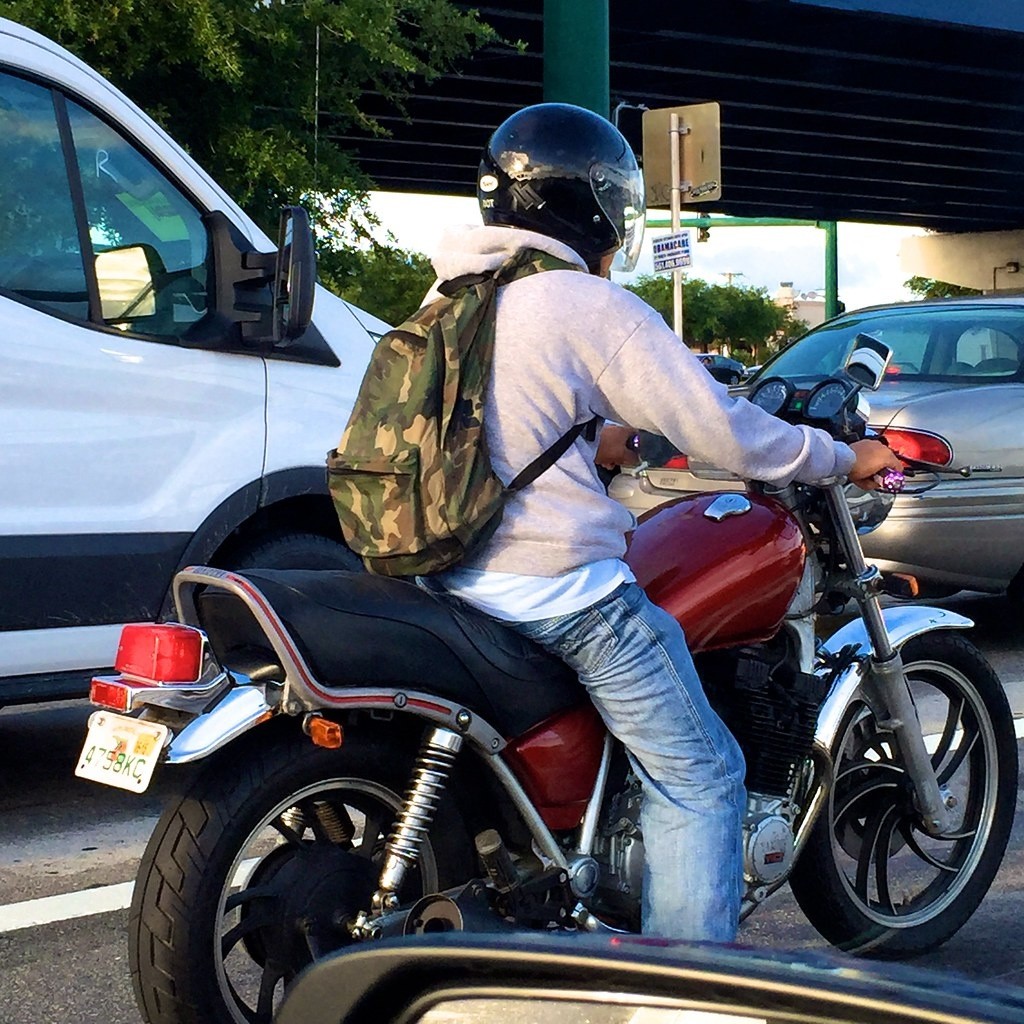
[741,365,763,380]
[606,297,1024,614]
[694,354,746,385]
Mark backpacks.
[323,255,599,576]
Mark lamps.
[1007,261,1019,274]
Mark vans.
[0,14,399,703]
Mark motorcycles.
[75,331,1017,1024]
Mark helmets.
[477,101,638,272]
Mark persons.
[419,104,902,942]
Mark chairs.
[951,353,1021,377]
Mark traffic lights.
[699,214,711,242]
